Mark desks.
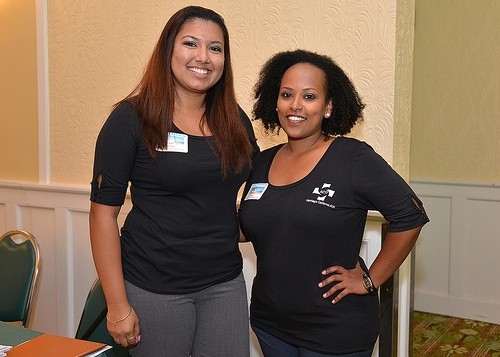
[0,320,113,357]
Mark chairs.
[75,277,113,346]
[0,230,43,329]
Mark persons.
[89,6,261,357]
[236,50,430,357]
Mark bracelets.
[106,307,132,323]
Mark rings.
[126,335,135,341]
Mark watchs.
[362,272,377,293]
